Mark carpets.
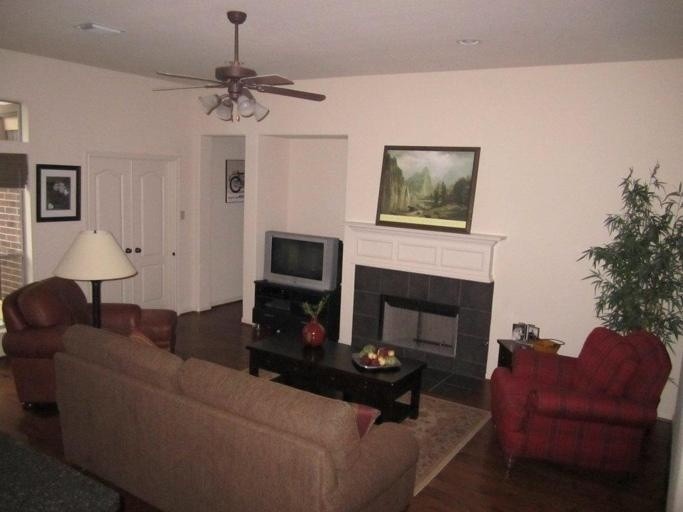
[228,367,495,499]
[0,424,116,512]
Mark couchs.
[58,327,419,512]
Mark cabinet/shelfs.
[251,280,342,341]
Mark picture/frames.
[35,162,83,223]
[374,146,481,234]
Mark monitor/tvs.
[263,231,343,292]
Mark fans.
[149,10,326,102]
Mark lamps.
[57,230,139,331]
[196,71,268,124]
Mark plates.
[349,352,402,371]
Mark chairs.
[1,277,179,418]
[485,326,673,483]
